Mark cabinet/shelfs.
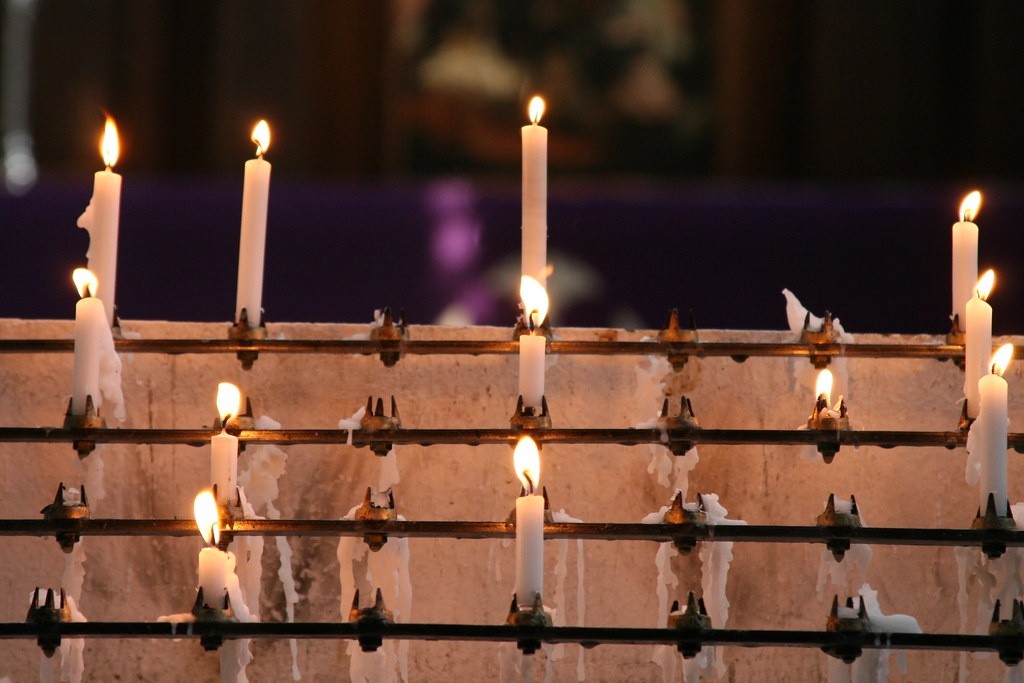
[0,338,1024,659]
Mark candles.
[72,296,102,408]
[980,373,1009,514]
[234,159,273,327]
[966,298,993,418]
[952,220,979,314]
[521,333,545,410]
[210,434,239,512]
[199,546,236,609]
[87,172,122,317]
[523,123,550,288]
[514,494,545,604]
